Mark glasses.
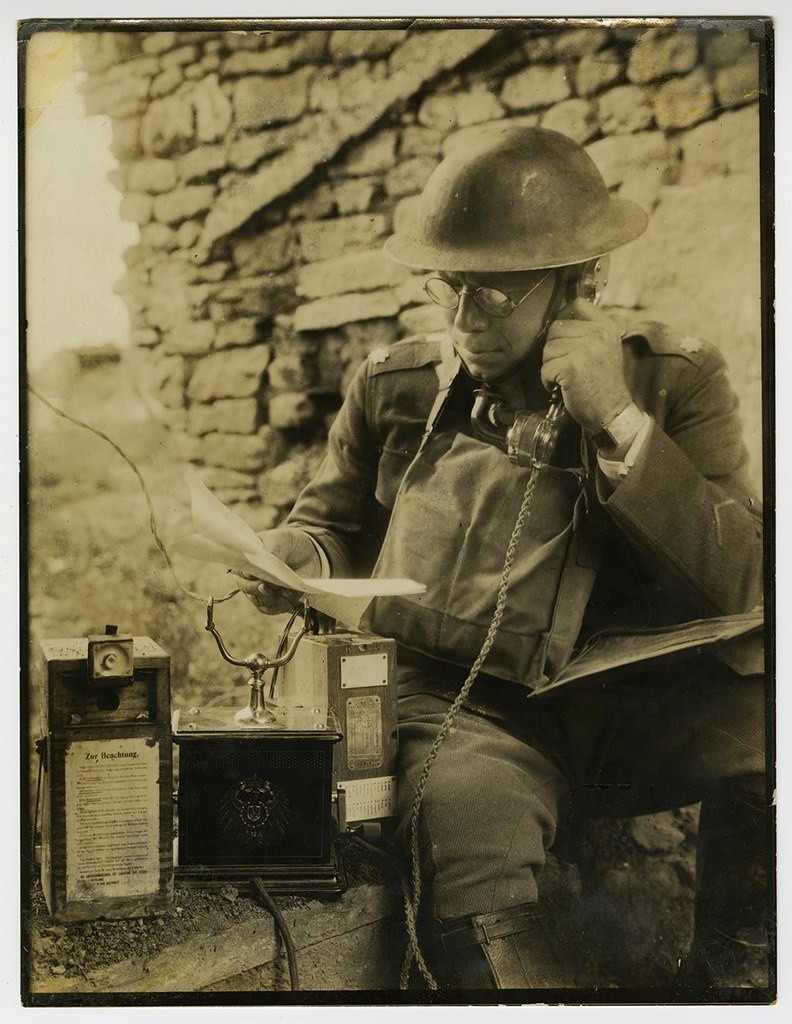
[423,270,548,320]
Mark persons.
[234,124,764,991]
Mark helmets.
[383,128,650,270]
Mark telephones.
[470,256,610,469]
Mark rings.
[253,596,263,606]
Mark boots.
[433,901,575,990]
[680,782,774,988]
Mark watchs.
[591,401,646,450]
